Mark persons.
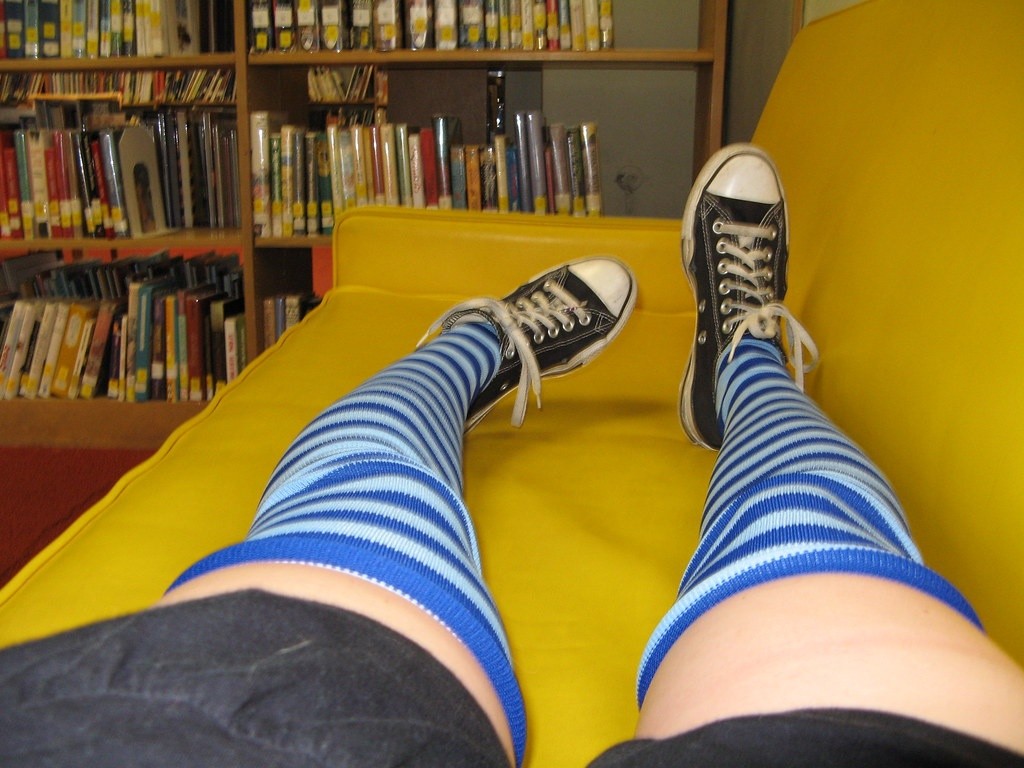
[1,137,1024,768]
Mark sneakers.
[679,143,819,453]
[415,254,638,439]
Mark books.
[3,244,326,401]
[0,0,214,57]
[248,110,609,236]
[302,63,376,102]
[0,63,245,240]
[244,0,618,54]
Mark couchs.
[0,0,1024,768]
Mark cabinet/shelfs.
[0,0,728,447]
